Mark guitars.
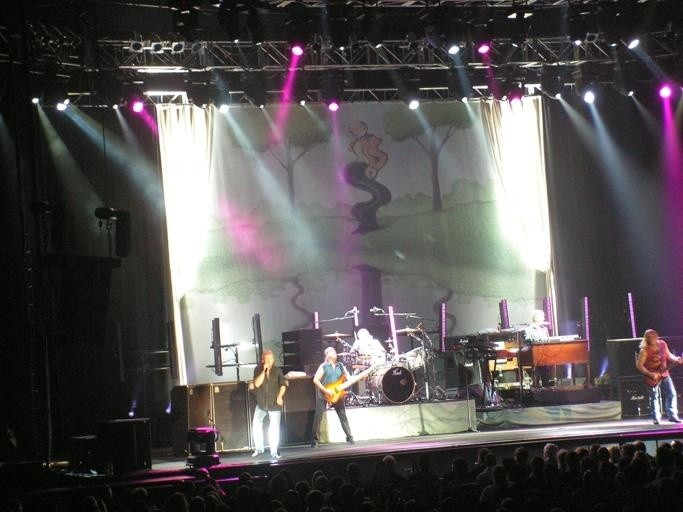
[643,357,683,387]
[321,365,375,404]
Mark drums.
[404,347,431,371]
[372,366,415,403]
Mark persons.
[638,328,683,424]
[350,328,387,356]
[251,350,286,458]
[311,346,356,449]
[529,310,550,339]
[83,441,683,512]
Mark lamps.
[128,37,640,58]
[34,76,679,113]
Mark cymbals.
[322,334,352,337]
[396,329,420,332]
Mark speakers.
[69,435,98,473]
[606,338,643,379]
[95,418,152,476]
[619,376,654,417]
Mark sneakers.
[311,441,318,449]
[271,454,282,459]
[668,416,682,423]
[653,419,660,424]
[346,436,356,444]
[252,449,263,457]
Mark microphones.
[265,368,267,372]
[540,322,550,327]
